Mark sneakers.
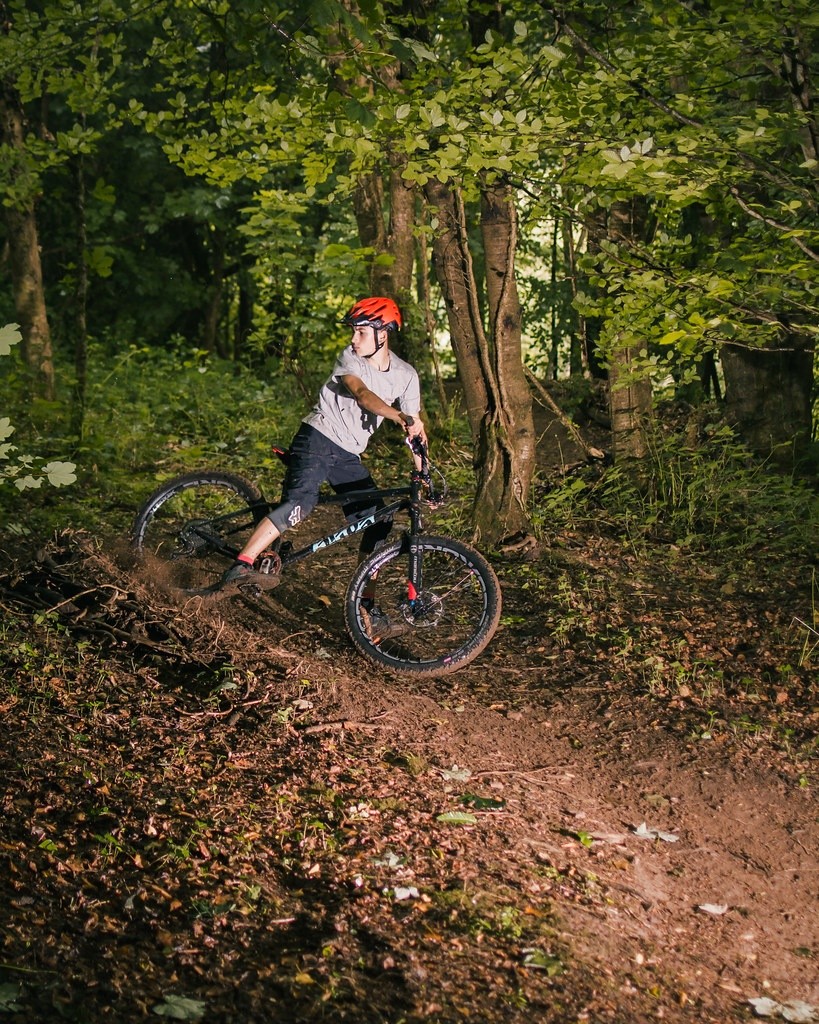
[359,606,410,638]
[220,566,280,591]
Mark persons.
[220,296,429,645]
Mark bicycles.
[128,415,503,679]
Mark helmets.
[344,297,401,335]
